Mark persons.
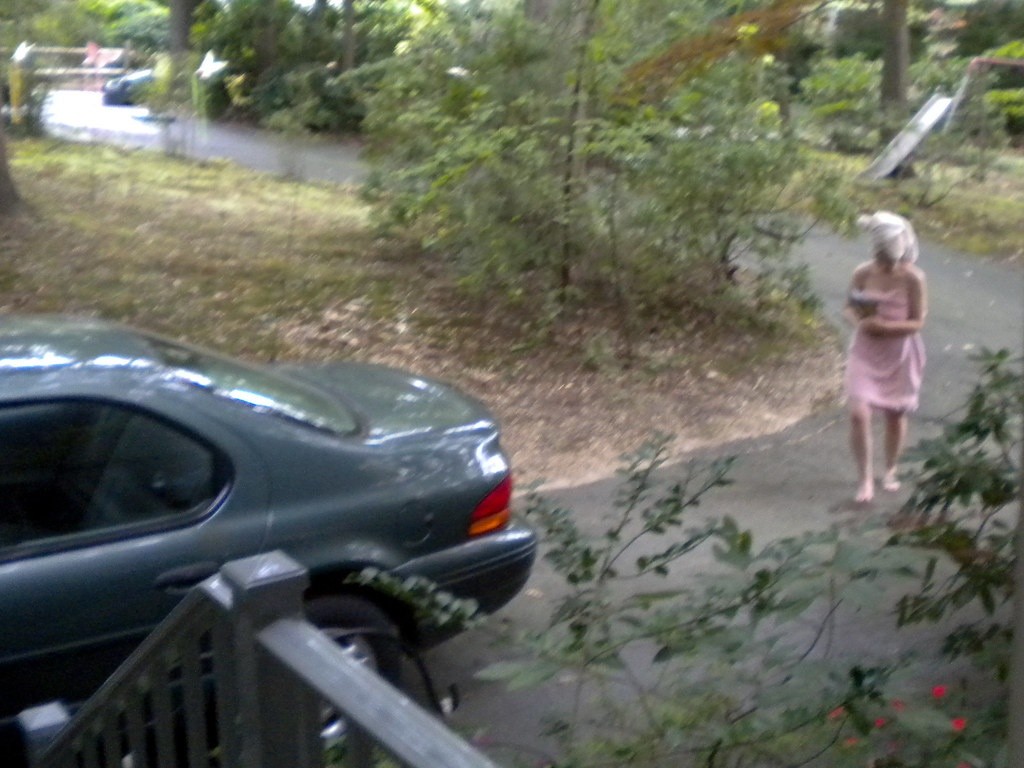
[846,212,927,501]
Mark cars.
[1,312,539,768]
[103,68,156,107]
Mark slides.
[852,96,954,188]
